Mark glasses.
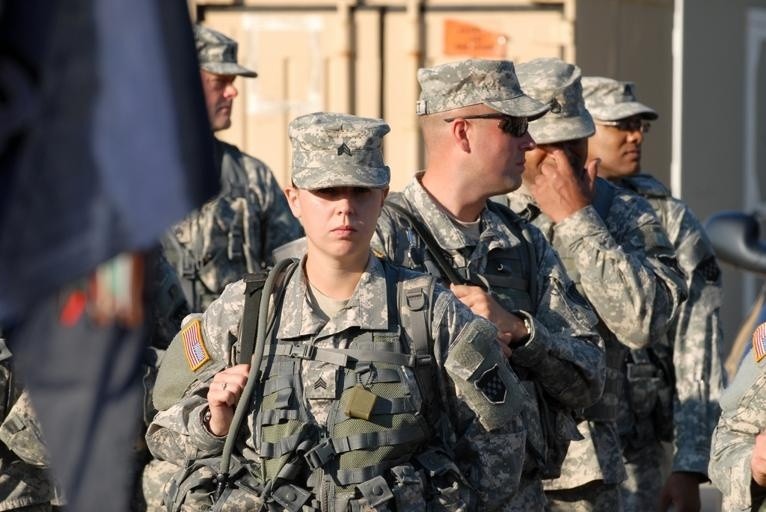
[593,119,651,133]
[444,113,528,137]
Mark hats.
[288,113,391,191]
[514,58,595,145]
[416,59,552,121]
[192,23,257,76]
[580,76,659,121]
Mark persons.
[369,58,608,512]
[160,25,306,315]
[581,72,725,512]
[0,338,160,512]
[141,109,532,512]
[0,1,222,512]
[512,57,689,512]
[707,322,766,512]
